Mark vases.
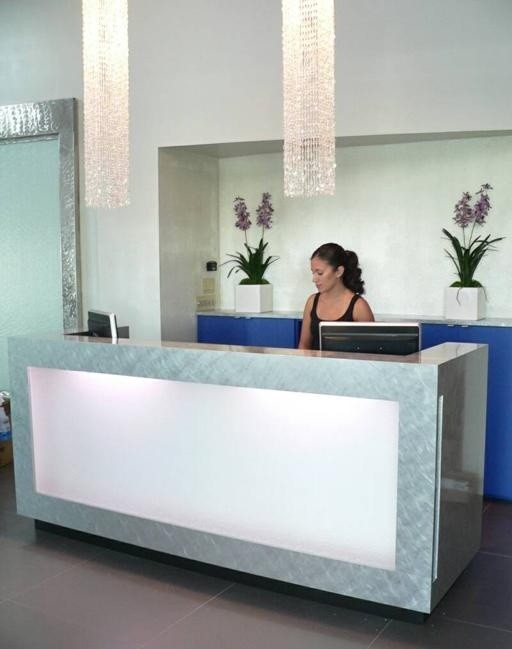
[233,284,275,314]
[442,287,489,322]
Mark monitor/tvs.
[319,321,422,354]
[87,310,118,338]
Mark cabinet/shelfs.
[195,313,302,349]
[421,323,511,504]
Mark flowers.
[217,191,281,285]
[440,182,508,305]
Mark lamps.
[80,0,133,209]
[280,0,337,199]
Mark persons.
[297,242,376,349]
